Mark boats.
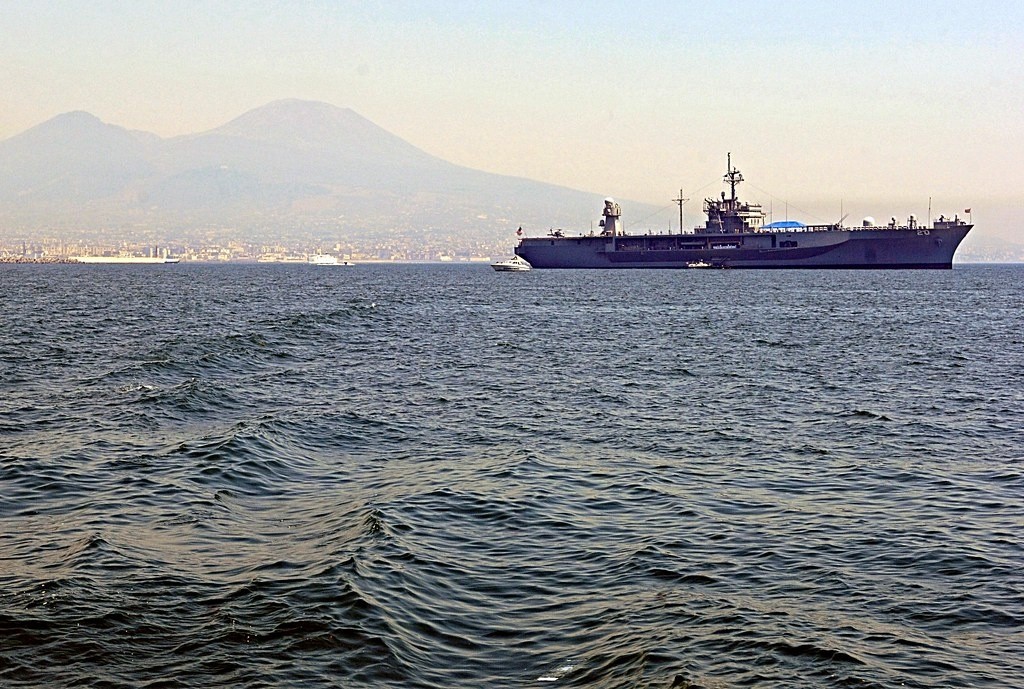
[491,256,530,272]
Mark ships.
[517,153,975,269]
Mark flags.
[964,208,971,213]
[515,227,523,235]
